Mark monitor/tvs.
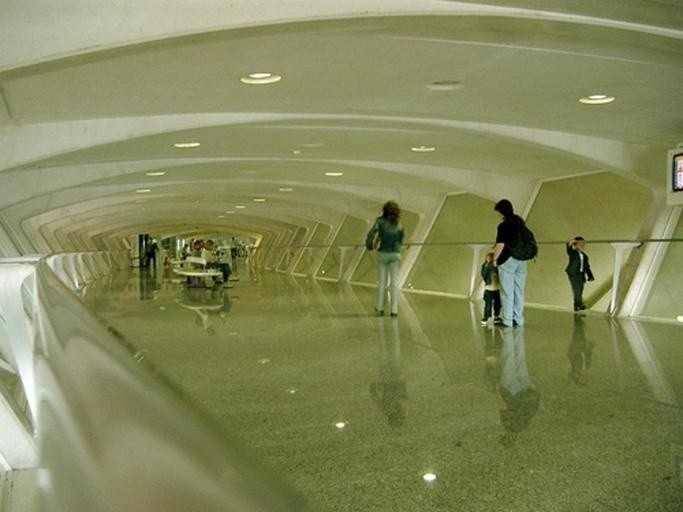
[666,143,683,207]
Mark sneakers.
[480,316,518,326]
[573,304,587,311]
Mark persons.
[481,253,502,325]
[496,327,539,446]
[140,233,247,289]
[493,199,525,327]
[565,236,595,314]
[366,201,405,318]
[139,257,255,336]
[566,317,596,387]
[480,327,504,395]
[367,319,410,432]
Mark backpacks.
[508,226,538,261]
